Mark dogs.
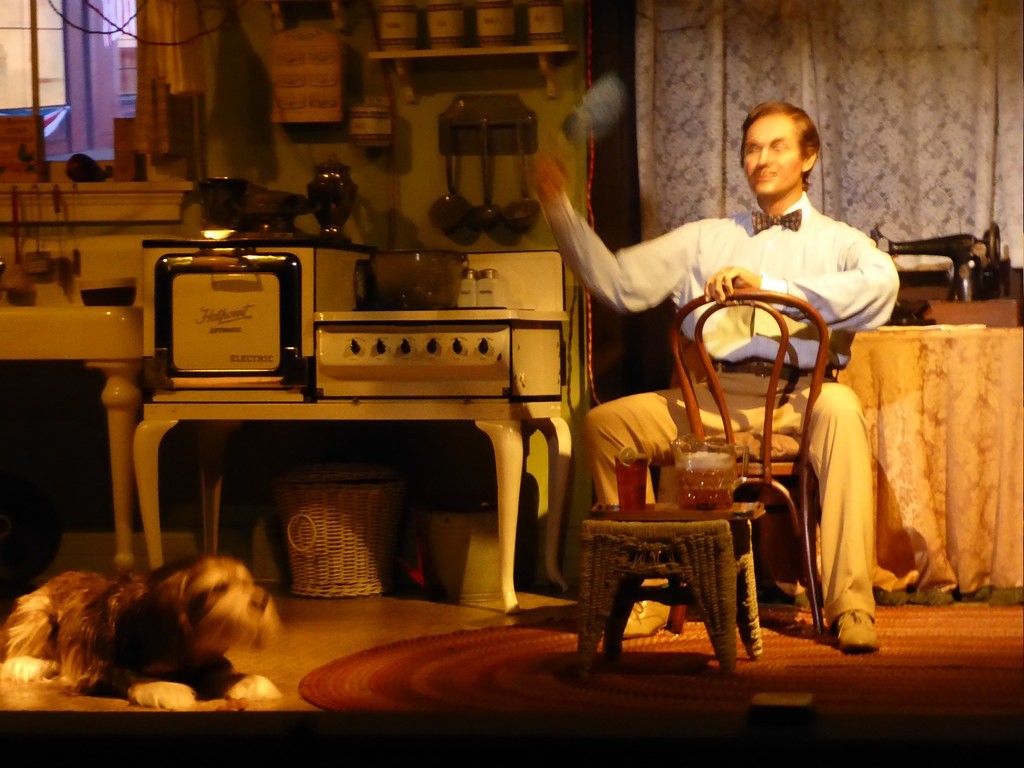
[0,552,284,704]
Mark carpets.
[298,605,1024,715]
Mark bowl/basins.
[79,276,137,306]
[372,248,468,310]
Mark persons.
[528,102,900,654]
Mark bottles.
[457,269,476,307]
[477,269,498,308]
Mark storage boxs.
[923,299,1019,326]
[112,117,185,182]
[0,115,48,183]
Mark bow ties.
[752,211,802,235]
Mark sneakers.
[837,610,880,652]
[623,598,672,639]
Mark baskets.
[276,471,403,597]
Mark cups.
[426,0,464,49]
[614,454,646,511]
[476,0,513,47]
[527,0,563,44]
[348,105,391,144]
[379,0,416,50]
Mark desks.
[133,402,572,616]
[0,307,144,575]
[829,327,1024,604]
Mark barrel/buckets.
[429,510,501,602]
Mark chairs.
[668,289,829,635]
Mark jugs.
[671,437,749,511]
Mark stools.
[579,519,764,676]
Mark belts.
[718,356,794,381]
